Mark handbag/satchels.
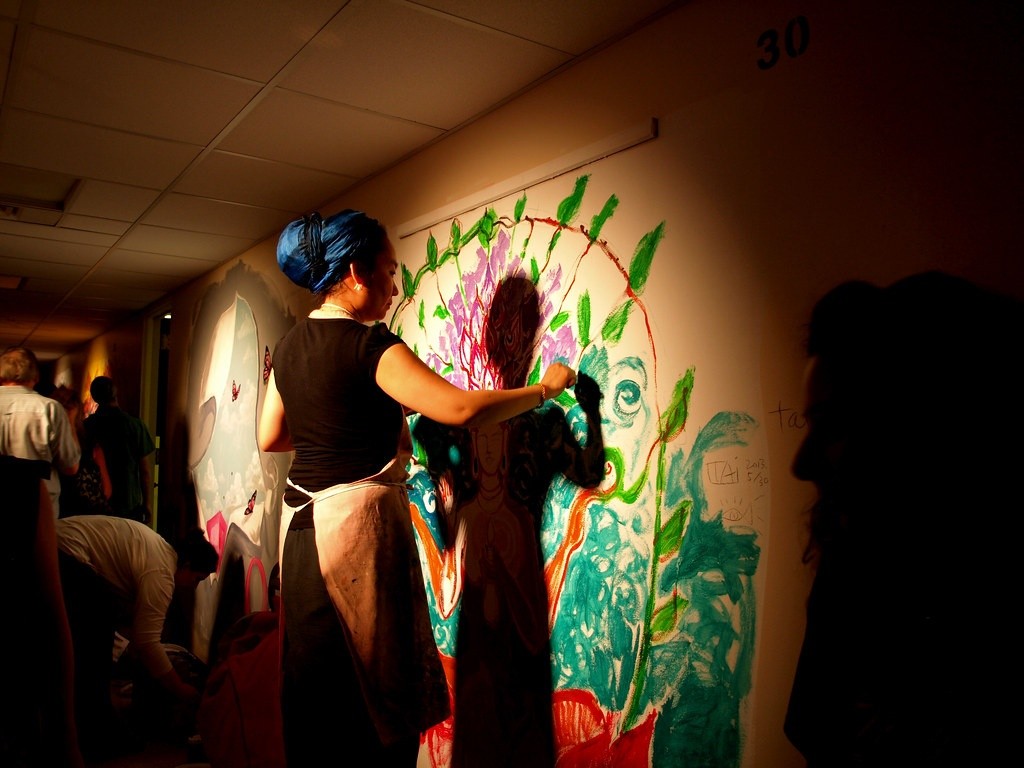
[74,458,105,511]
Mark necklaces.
[321,303,361,323]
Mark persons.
[781,270,1024,768]
[258,207,577,768]
[0,345,155,525]
[56,515,219,765]
[0,454,86,768]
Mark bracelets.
[535,383,546,409]
